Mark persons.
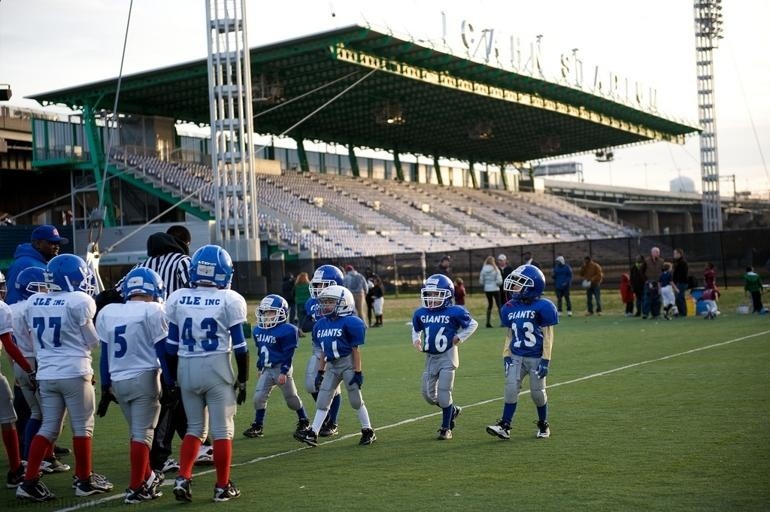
[0,223,251,503]
[453,277,465,306]
[242,293,309,439]
[486,264,551,441]
[479,256,503,328]
[411,273,479,440]
[620,247,688,320]
[743,265,762,314]
[496,254,513,304]
[521,252,541,269]
[304,264,345,438]
[293,285,377,447]
[552,256,573,317]
[701,263,720,320]
[580,256,604,316]
[281,264,385,338]
[438,256,451,279]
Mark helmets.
[121,267,167,303]
[14,254,96,300]
[0,273,7,300]
[188,245,234,289]
[502,265,546,299]
[309,264,355,318]
[419,273,455,310]
[255,293,290,330]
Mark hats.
[498,254,507,261]
[31,224,68,246]
[443,255,452,262]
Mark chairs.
[110,153,641,259]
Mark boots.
[486,310,495,328]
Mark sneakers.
[124,445,241,504]
[487,419,511,440]
[7,446,114,503]
[567,311,603,316]
[244,422,263,438]
[294,418,338,441]
[359,427,376,446]
[436,406,462,439]
[533,420,550,438]
[624,313,648,319]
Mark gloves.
[535,359,550,380]
[162,381,180,409]
[315,370,326,392]
[349,372,363,389]
[233,378,247,405]
[504,357,514,377]
[97,385,118,417]
